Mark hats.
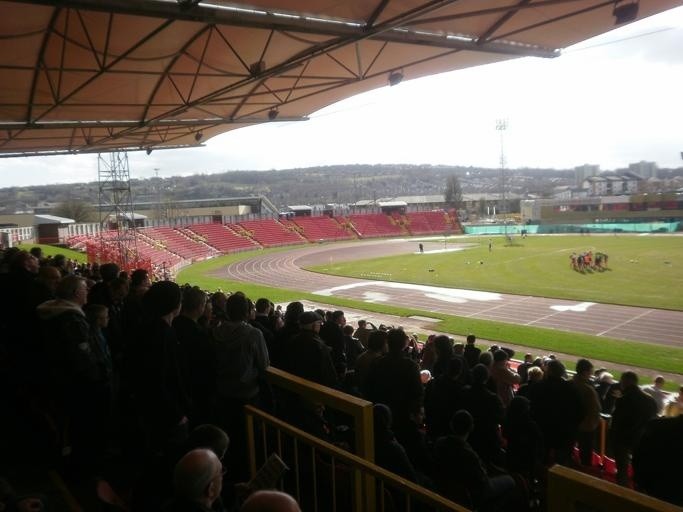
[300,312,323,324]
[146,281,181,316]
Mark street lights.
[495,121,507,237]
[152,168,159,229]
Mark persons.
[2,234,681,510]
